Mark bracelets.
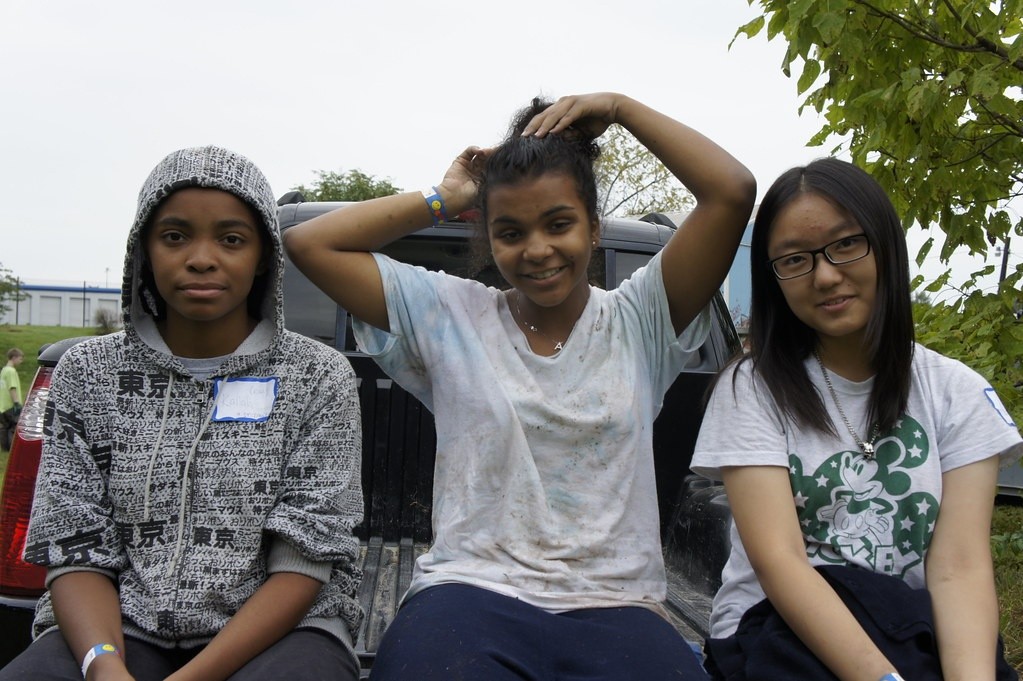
[82,644,120,677]
[880,672,906,681]
[422,185,446,227]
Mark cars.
[0,193,756,679]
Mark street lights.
[106,267,109,288]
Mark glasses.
[767,232,870,280]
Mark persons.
[0,348,24,451]
[282,93,757,681]
[689,159,1023,681]
[0,146,365,681]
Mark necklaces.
[815,351,877,459]
[516,292,563,350]
